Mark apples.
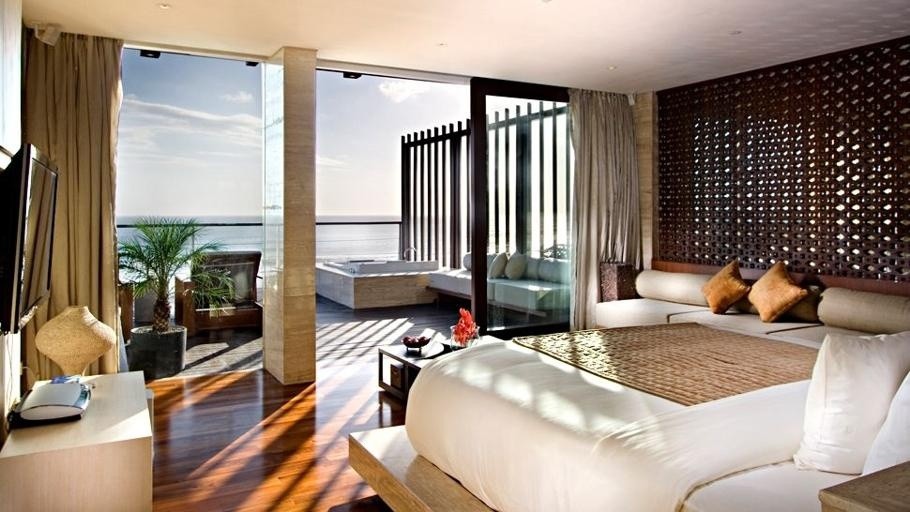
[401,336,427,344]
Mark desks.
[818,461,910,512]
[377,334,507,400]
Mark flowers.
[451,305,480,345]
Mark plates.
[400,338,431,348]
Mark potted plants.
[117,215,237,381]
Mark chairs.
[175,248,263,337]
[107,274,136,345]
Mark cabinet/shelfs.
[1,371,155,512]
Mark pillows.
[487,250,527,281]
[702,260,749,313]
[792,330,908,475]
[637,269,711,307]
[746,261,808,322]
[815,288,907,335]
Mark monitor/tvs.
[0,141,61,334]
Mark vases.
[448,323,480,351]
[35,305,114,376]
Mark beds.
[348,261,909,512]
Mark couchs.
[430,251,571,322]
